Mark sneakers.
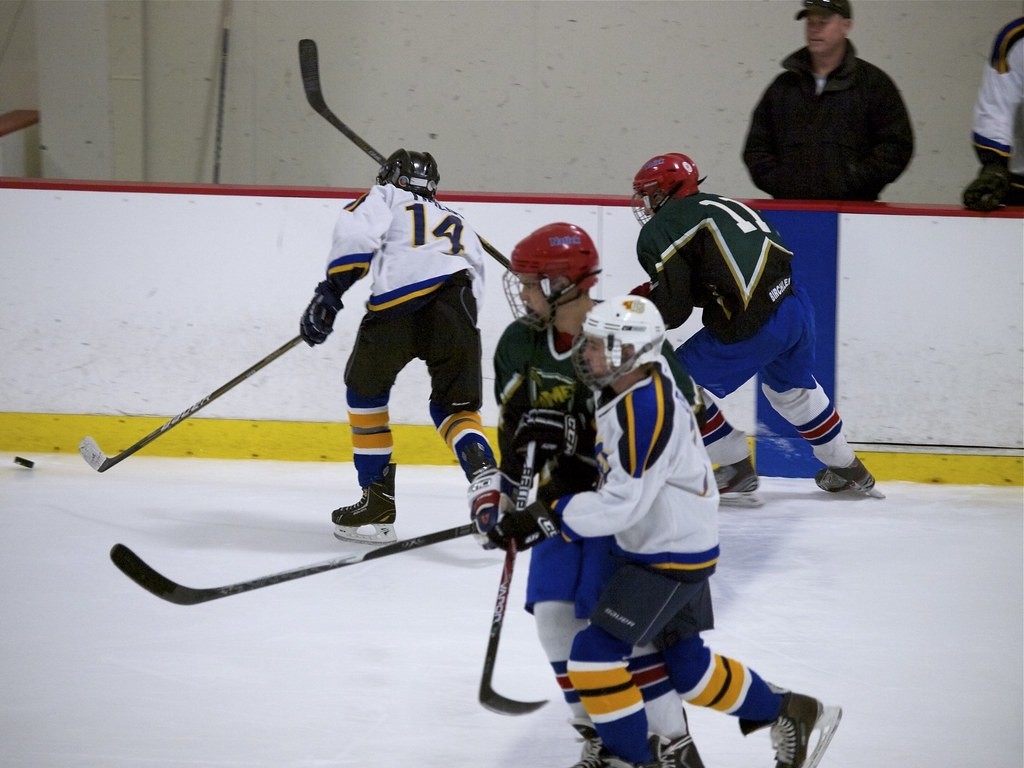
[569,720,607,768]
[332,462,397,543]
[594,734,664,768]
[712,456,766,507]
[660,706,706,768]
[815,456,887,499]
[740,682,843,768]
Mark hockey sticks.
[110,514,480,606]
[478,442,550,716]
[297,37,513,273]
[77,335,305,473]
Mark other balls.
[14,457,34,468]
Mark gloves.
[469,468,522,550]
[628,281,651,298]
[299,282,343,347]
[486,501,559,552]
[962,173,1011,211]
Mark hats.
[796,0,852,20]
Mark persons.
[742,0,913,203]
[300,147,496,551]
[627,153,885,507]
[961,17,1024,210]
[481,222,843,768]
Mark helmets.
[631,153,700,228]
[503,222,602,331]
[377,148,441,200]
[571,294,666,387]
